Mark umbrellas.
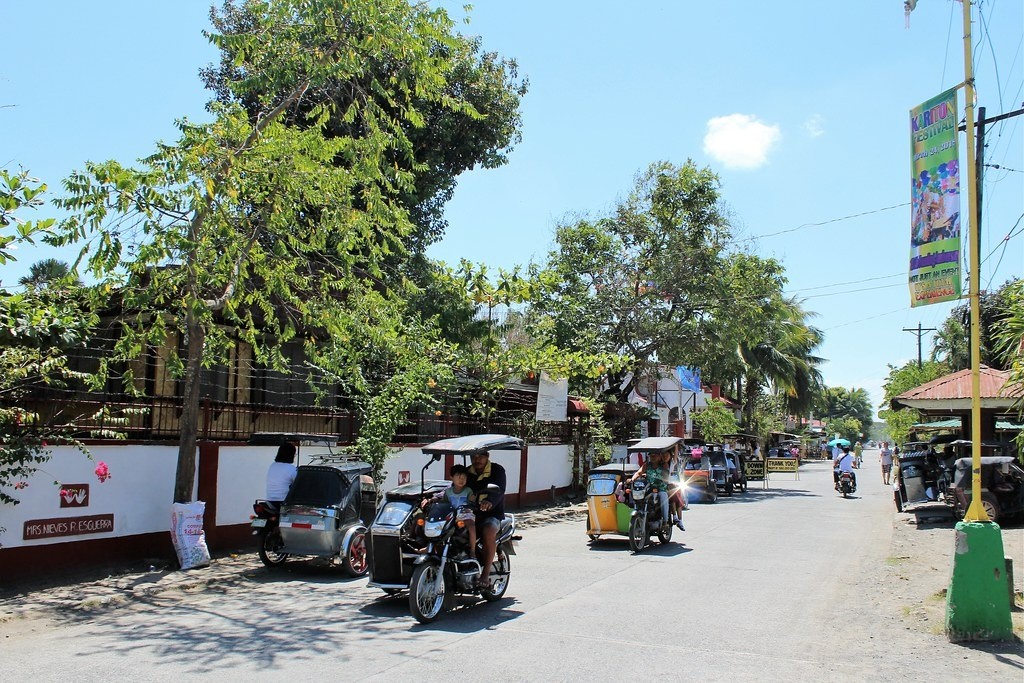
[827,439,852,447]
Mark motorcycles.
[361,434,528,624]
[704,441,733,497]
[949,456,1024,522]
[584,436,686,554]
[251,456,380,578]
[676,438,717,505]
[836,471,856,498]
[725,451,747,492]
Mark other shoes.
[673,519,681,523]
[676,524,685,531]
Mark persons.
[912,440,962,479]
[656,441,686,533]
[264,441,299,506]
[867,439,885,450]
[833,446,858,491]
[892,442,902,467]
[688,433,830,476]
[614,474,635,509]
[878,441,895,485]
[629,450,670,532]
[443,464,481,571]
[852,441,863,466]
[832,444,845,485]
[420,445,507,591]
